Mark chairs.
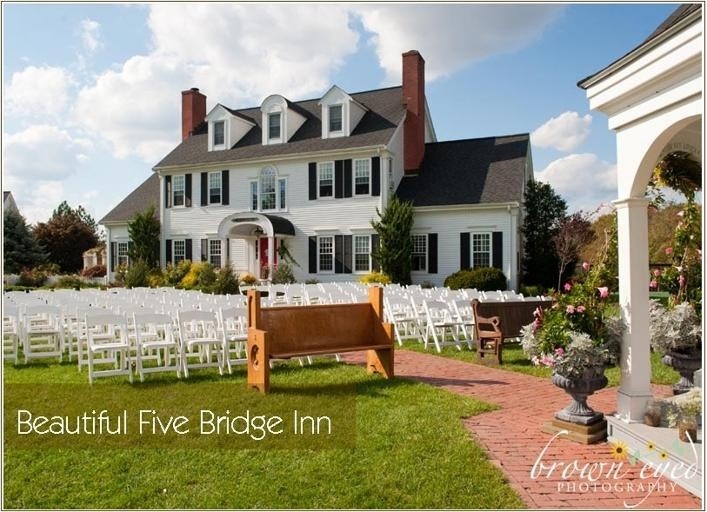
[4,280,556,394]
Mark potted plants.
[679,403,700,442]
[644,398,662,427]
[653,301,702,392]
[549,329,616,416]
